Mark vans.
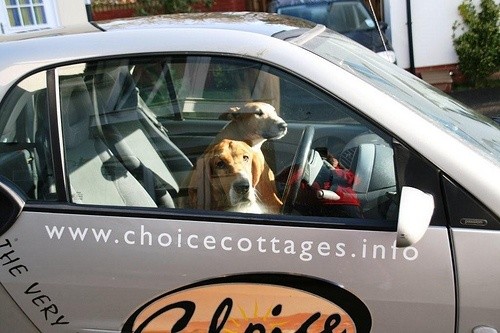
[269,0,396,64]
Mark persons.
[302,155,365,220]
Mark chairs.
[50,76,176,209]
[96,59,195,196]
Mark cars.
[0,11,500,332]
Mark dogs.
[188,103,288,214]
